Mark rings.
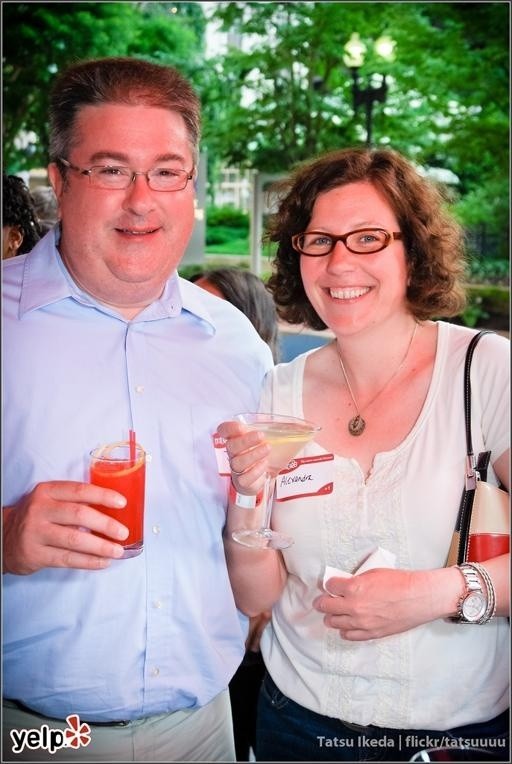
[228,460,246,475]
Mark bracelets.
[228,477,265,509]
[461,561,497,626]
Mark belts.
[4,698,130,727]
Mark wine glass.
[230,412,319,552]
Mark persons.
[188,267,280,366]
[3,174,42,260]
[1,57,276,762]
[217,144,511,761]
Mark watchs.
[450,564,487,625]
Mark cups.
[83,444,147,561]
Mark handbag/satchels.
[439,330,511,624]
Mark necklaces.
[333,317,419,436]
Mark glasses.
[291,227,403,257]
[58,157,196,193]
